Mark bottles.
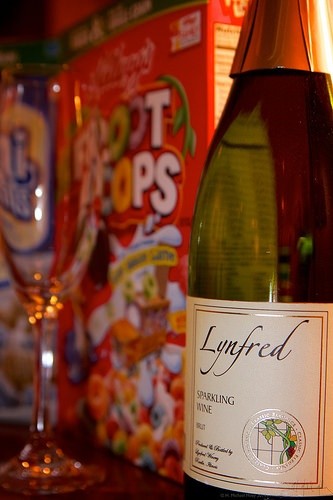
[182,0,333,500]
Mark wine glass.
[0,62,108,496]
